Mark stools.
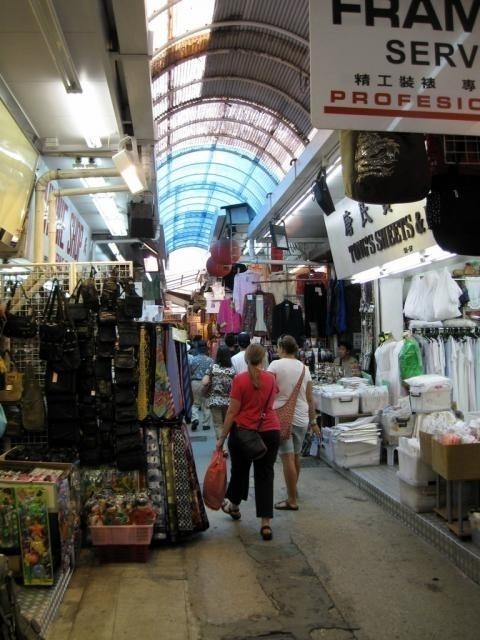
[381,444,398,466]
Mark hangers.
[237,264,260,276]
[280,295,292,306]
[411,326,480,344]
[252,283,267,295]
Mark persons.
[266,333,321,511]
[374,331,400,407]
[399,332,423,393]
[230,332,250,373]
[334,340,361,376]
[226,333,240,356]
[215,342,282,540]
[202,345,238,457]
[190,345,214,430]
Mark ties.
[135,324,193,425]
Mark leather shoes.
[203,425,210,430]
[191,419,199,430]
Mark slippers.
[221,500,241,520]
[261,526,272,540]
[274,499,298,510]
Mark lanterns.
[206,257,232,277]
[210,239,241,264]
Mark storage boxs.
[312,387,480,514]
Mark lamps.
[312,167,336,216]
[111,136,148,194]
[30,0,82,94]
[269,220,289,250]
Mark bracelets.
[309,418,318,427]
[219,435,227,440]
[222,429,228,435]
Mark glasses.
[280,334,286,342]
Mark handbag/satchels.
[0,349,25,402]
[203,446,228,511]
[3,281,37,338]
[21,362,47,433]
[275,407,293,440]
[237,429,266,459]
[39,267,145,471]
[301,431,319,457]
[199,362,213,399]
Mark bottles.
[309,434,319,456]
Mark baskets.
[89,524,153,545]
[90,546,149,562]
